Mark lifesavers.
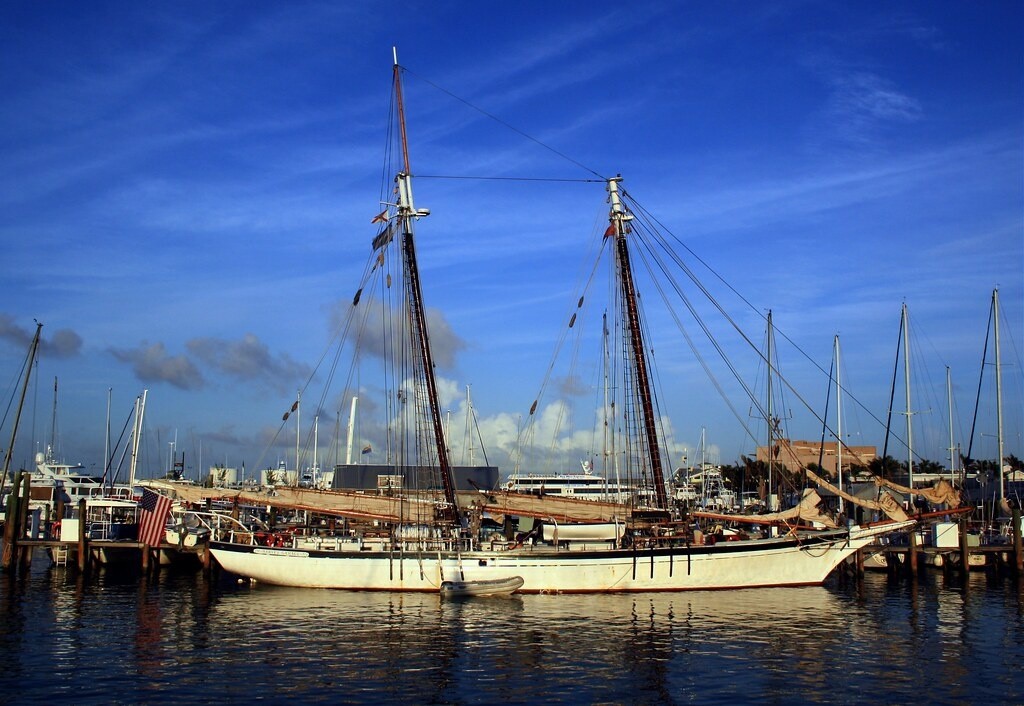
[727,536,742,541]
[286,527,300,535]
[53,522,61,536]
[267,532,282,547]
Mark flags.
[372,221,393,252]
[137,487,174,549]
[371,210,388,223]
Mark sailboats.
[202,45,919,595]
[0,282,1024,575]
